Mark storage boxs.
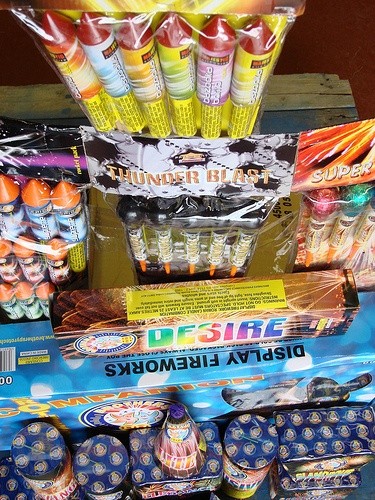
[48,268,360,359]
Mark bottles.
[40,10,274,106]
[154,404,206,479]
[1,175,86,320]
[220,372,372,410]
